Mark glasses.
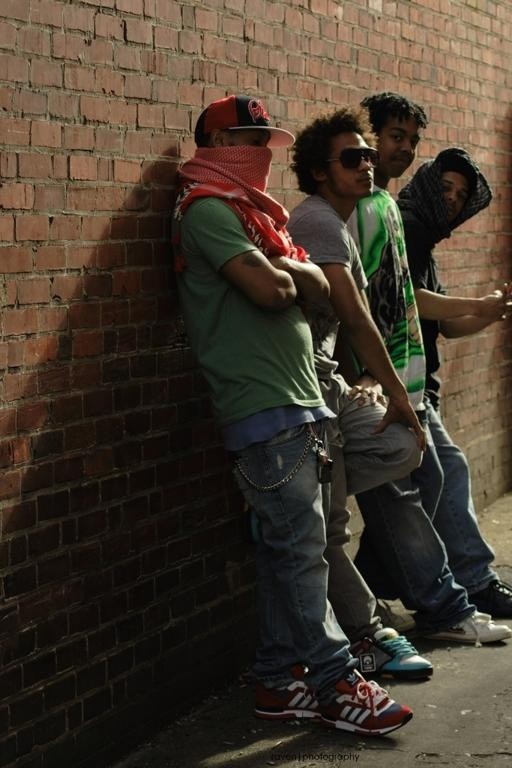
[324,147,381,170]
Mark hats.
[194,93,296,149]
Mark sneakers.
[250,664,322,722]
[315,667,413,738]
[350,624,435,680]
[468,577,512,616]
[422,608,511,648]
[375,596,418,633]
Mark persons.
[168,92,413,736]
[285,104,432,680]
[393,146,510,616]
[335,93,512,648]
